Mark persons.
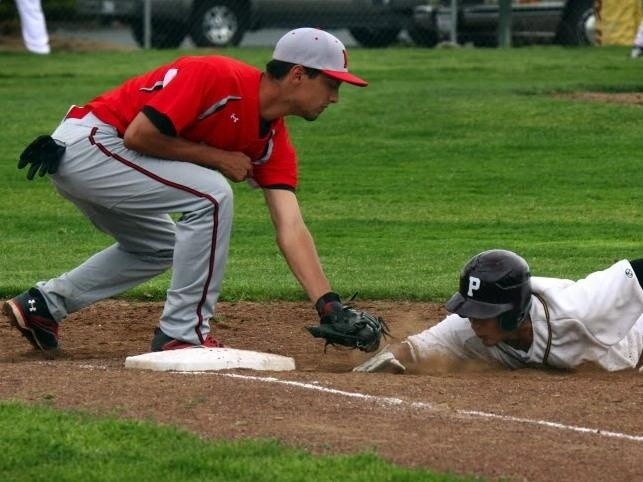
[15,0,52,56]
[2,27,382,358]
[351,249,642,374]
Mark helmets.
[444,249,533,329]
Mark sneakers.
[2,287,60,353]
[150,326,230,352]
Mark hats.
[272,26,370,88]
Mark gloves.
[351,343,406,374]
[17,134,68,181]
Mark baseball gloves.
[307,293,383,353]
